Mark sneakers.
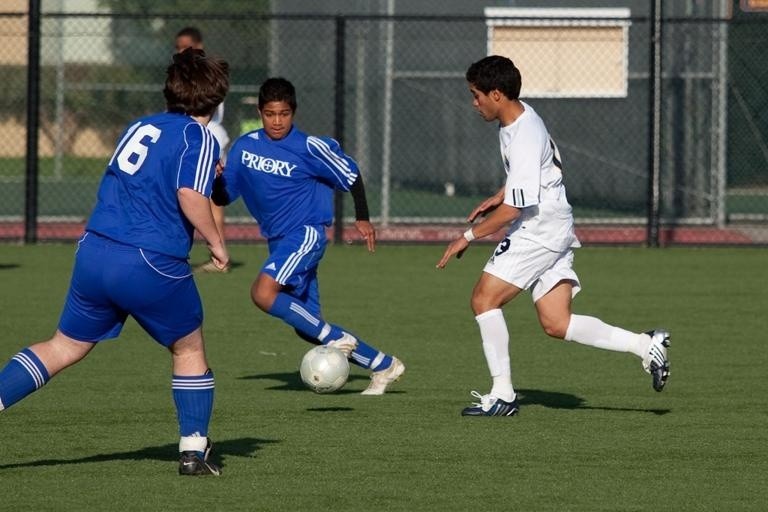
[640,329,672,393]
[177,436,222,477]
[461,390,520,417]
[199,262,228,273]
[324,331,360,359]
[361,355,406,395]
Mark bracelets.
[462,227,476,243]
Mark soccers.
[299,345,349,393]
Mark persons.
[435,53,671,421]
[212,75,408,396]
[172,26,232,275]
[0,45,235,477]
